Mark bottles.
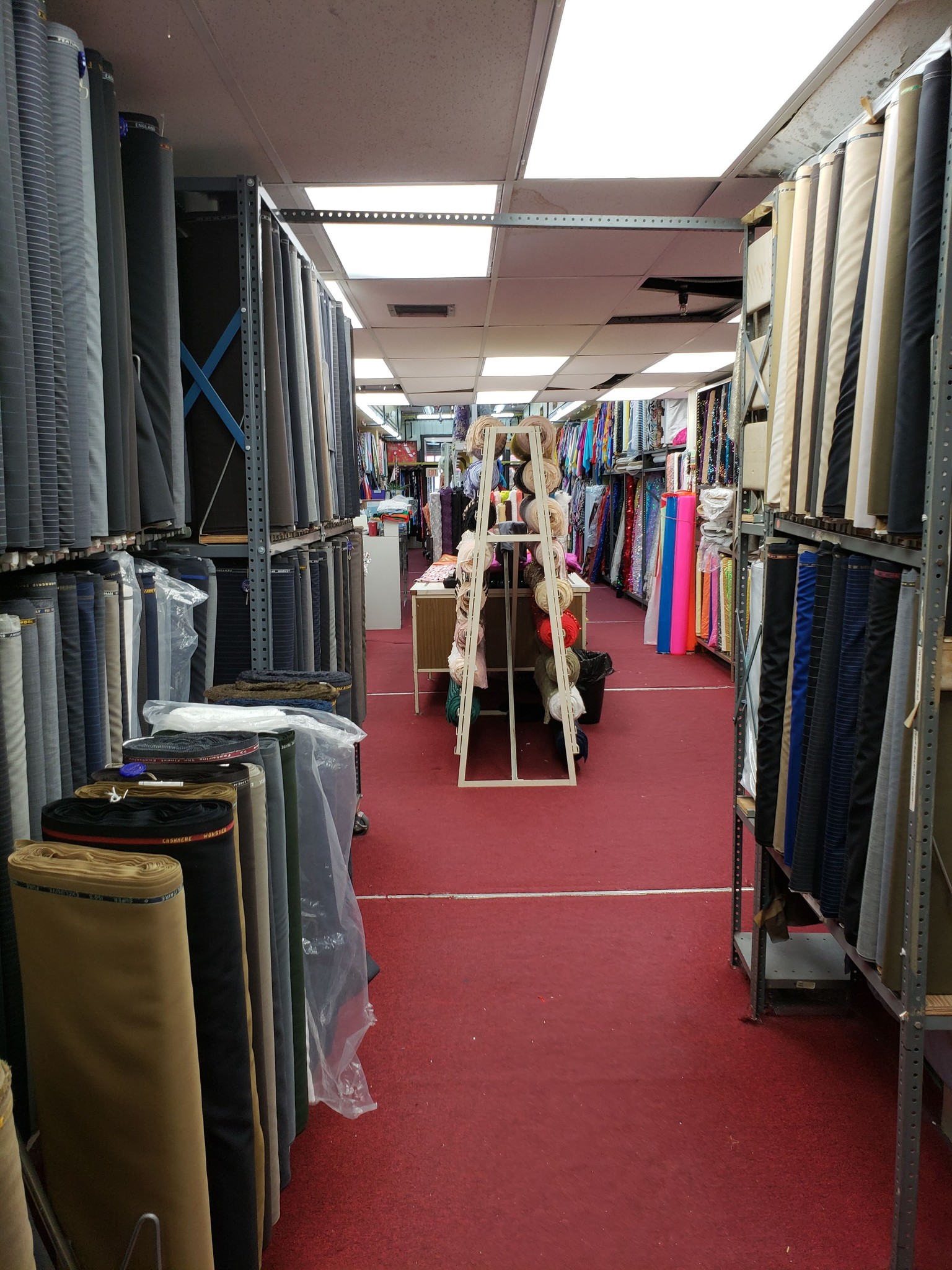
[379,523,384,537]
[456,468,462,488]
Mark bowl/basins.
[370,520,382,529]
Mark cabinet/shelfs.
[599,444,686,605]
[176,173,368,836]
[731,25,952,1270]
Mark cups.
[368,522,378,536]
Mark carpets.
[264,552,952,1270]
